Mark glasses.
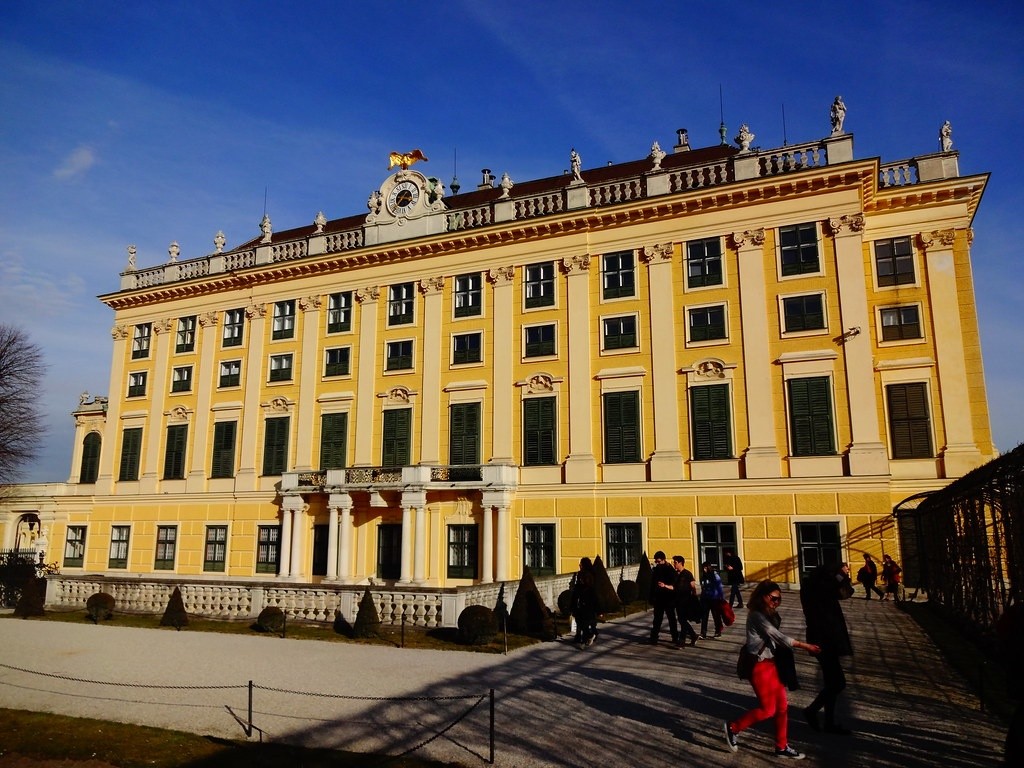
[769,596,781,602]
[654,560,661,563]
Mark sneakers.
[724,723,738,752]
[778,745,805,760]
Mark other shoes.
[676,642,686,647]
[880,592,884,600]
[691,634,699,644]
[580,644,585,649]
[715,632,721,636]
[698,635,706,639]
[588,634,596,646]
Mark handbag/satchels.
[857,567,867,582]
[721,600,735,626]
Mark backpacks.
[736,643,758,681]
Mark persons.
[940,119,953,151]
[881,555,902,601]
[830,96,847,133]
[129,246,136,266]
[646,547,743,649]
[570,151,582,180]
[802,552,855,736]
[570,558,598,647]
[858,554,884,601]
[724,581,821,761]
[998,671,1024,768]
